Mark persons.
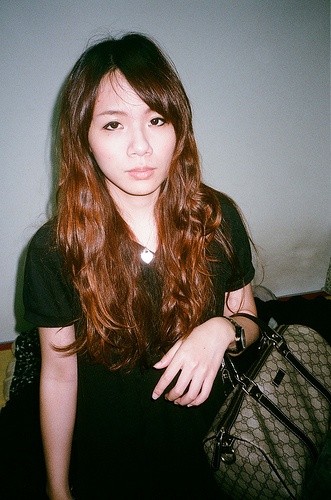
[24,31,263,500]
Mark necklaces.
[125,221,167,266]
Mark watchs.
[218,313,248,355]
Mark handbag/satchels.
[201,313,331,500]
